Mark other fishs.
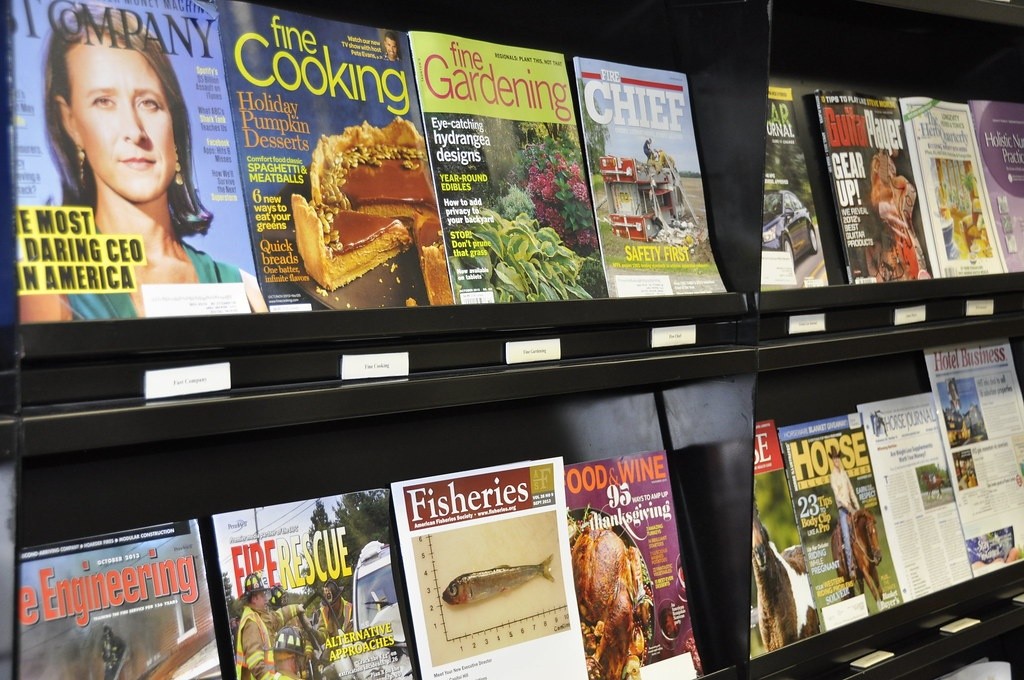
[442,552,556,606]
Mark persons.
[382,31,402,62]
[19,5,269,324]
[234,571,352,680]
[827,445,862,581]
[642,137,653,159]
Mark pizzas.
[290,113,452,307]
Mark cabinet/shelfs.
[0,0,1024,680]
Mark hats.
[829,446,841,458]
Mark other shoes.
[850,570,857,582]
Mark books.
[760,87,1024,293]
[388,449,705,680]
[16,518,222,679]
[206,488,416,680]
[749,335,1024,660]
[7,0,731,324]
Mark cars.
[354,540,407,651]
[761,189,819,273]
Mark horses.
[831,508,883,613]
[921,472,945,498]
[752,499,821,652]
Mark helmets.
[266,626,306,657]
[321,579,345,606]
[240,573,273,599]
[269,585,288,608]
